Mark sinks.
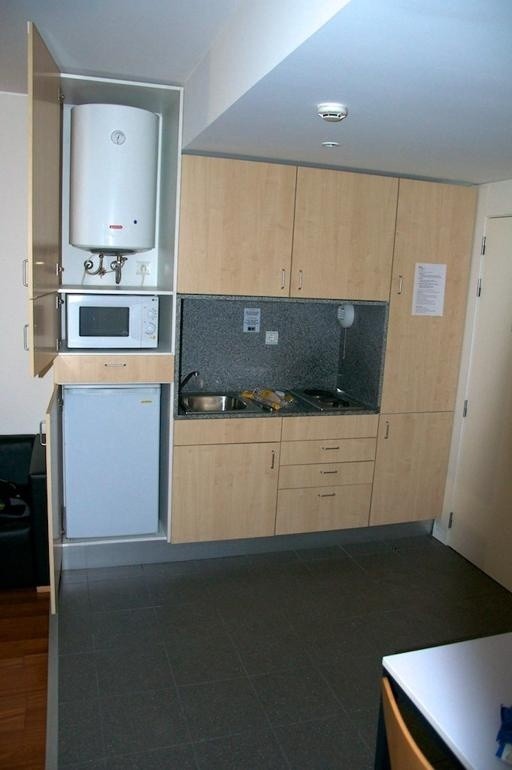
[180,393,263,414]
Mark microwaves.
[66,294,158,351]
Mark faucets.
[180,371,200,392]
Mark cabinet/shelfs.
[22,72,181,547]
[380,179,479,410]
[180,154,398,301]
[175,409,454,545]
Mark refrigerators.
[57,383,160,539]
